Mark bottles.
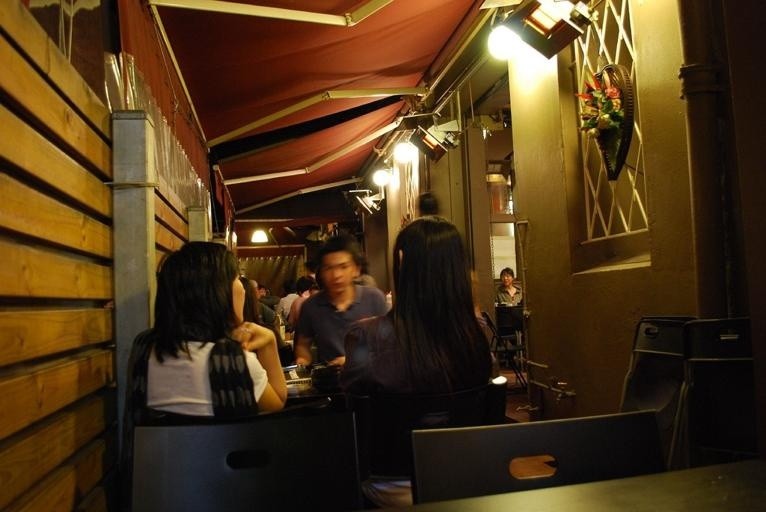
[272,311,286,343]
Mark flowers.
[572,62,626,140]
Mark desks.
[397,460,766,512]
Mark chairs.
[126,370,667,511]
[481,302,529,394]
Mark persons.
[240,231,389,367]
[495,268,524,307]
[339,215,497,507]
[400,191,448,234]
[123,241,289,510]
